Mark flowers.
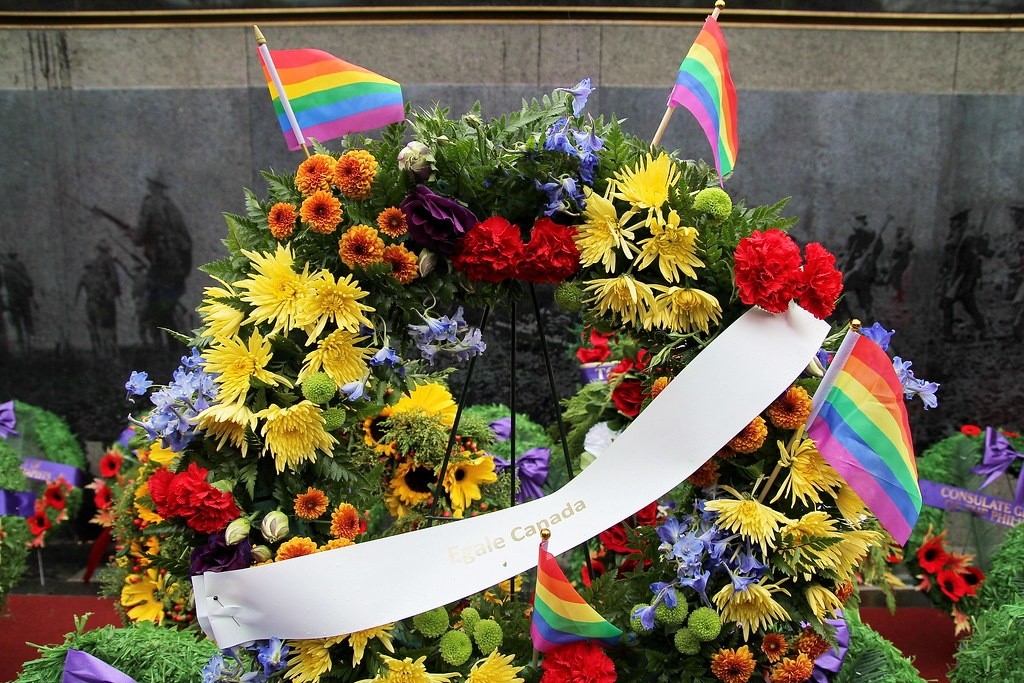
[0,77,1024,683]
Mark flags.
[530,541,626,652]
[257,46,404,152]
[803,330,922,547]
[668,16,739,190]
